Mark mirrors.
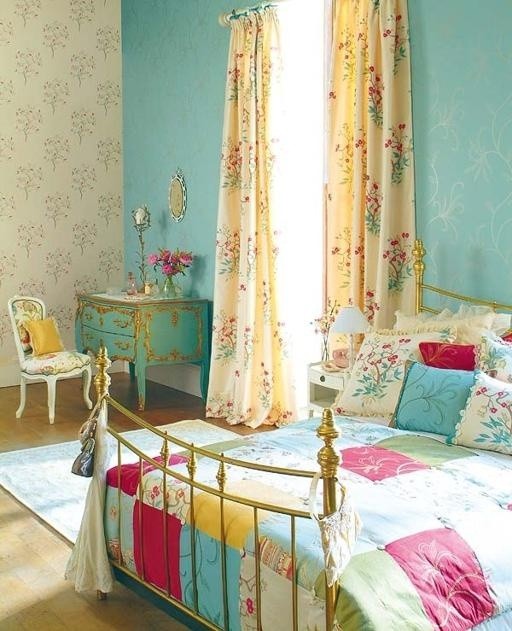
[168,168,187,224]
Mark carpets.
[0,418,242,545]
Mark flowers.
[148,247,194,286]
[310,315,336,361]
[130,205,152,294]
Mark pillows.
[331,303,512,457]
[23,315,64,357]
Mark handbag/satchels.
[71,438,95,477]
[309,473,363,587]
[79,391,108,444]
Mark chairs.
[7,294,92,424]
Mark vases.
[320,334,332,366]
[161,275,176,299]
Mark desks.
[75,291,210,412]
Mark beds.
[93,241,512,631]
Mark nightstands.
[308,361,352,417]
